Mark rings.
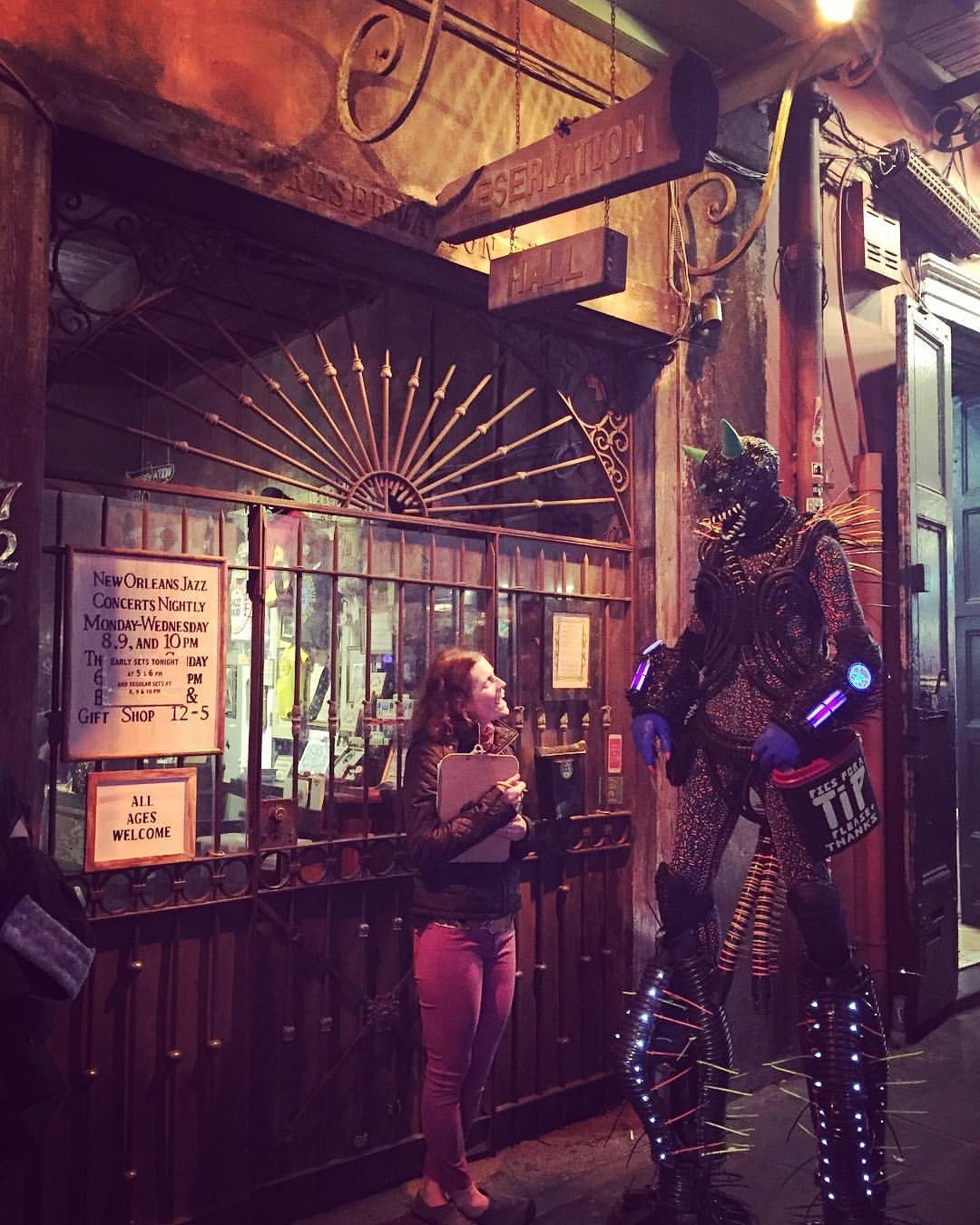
[508,829,509,834]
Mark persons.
[403,647,537,1225]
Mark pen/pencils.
[497,781,528,792]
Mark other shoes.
[412,1191,476,1225]
[463,1187,534,1225]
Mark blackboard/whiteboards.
[63,545,226,763]
[84,768,196,873]
